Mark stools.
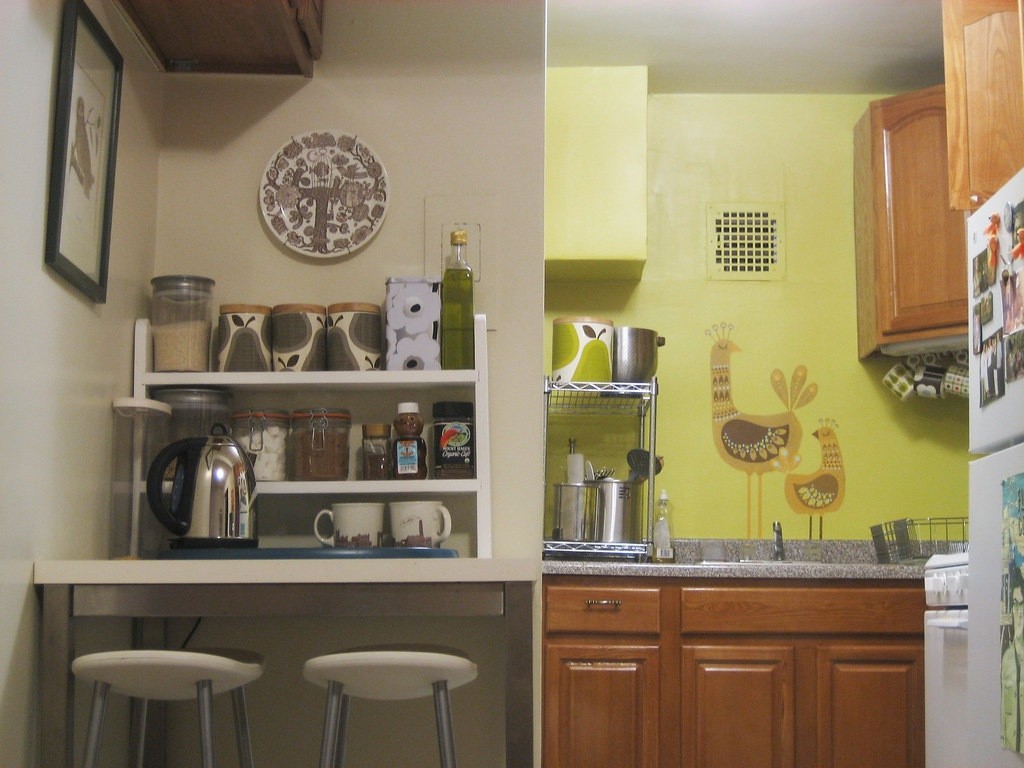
[71,645,267,768]
[301,649,478,768]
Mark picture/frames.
[46,0,125,304]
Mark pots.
[612,326,665,392]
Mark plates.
[258,128,391,258]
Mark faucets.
[773,522,786,562]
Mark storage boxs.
[385,276,443,370]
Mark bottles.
[112,398,171,561]
[652,488,674,564]
[231,409,287,482]
[393,402,427,480]
[151,390,231,477]
[363,422,387,480]
[441,230,473,370]
[151,276,215,371]
[291,408,351,481]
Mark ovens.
[924,565,969,768]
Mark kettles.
[147,422,255,547]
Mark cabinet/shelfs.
[541,374,658,564]
[941,0,1024,212]
[114,317,491,559]
[118,0,324,79]
[853,84,967,362]
[540,574,927,768]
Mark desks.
[33,553,541,768]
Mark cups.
[389,501,452,547]
[882,354,920,401]
[218,302,380,371]
[314,502,387,547]
[943,350,969,400]
[552,315,614,389]
[553,483,599,541]
[584,479,643,543]
[913,353,948,400]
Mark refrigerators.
[966,166,1024,768]
